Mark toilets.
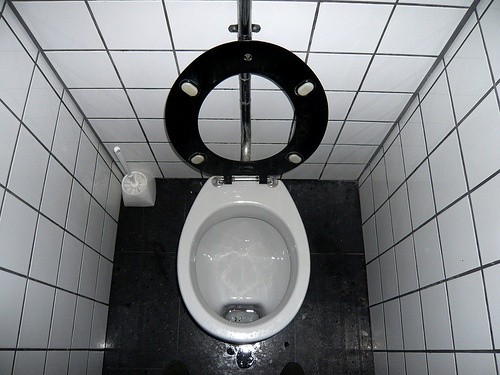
[165,42,329,342]
